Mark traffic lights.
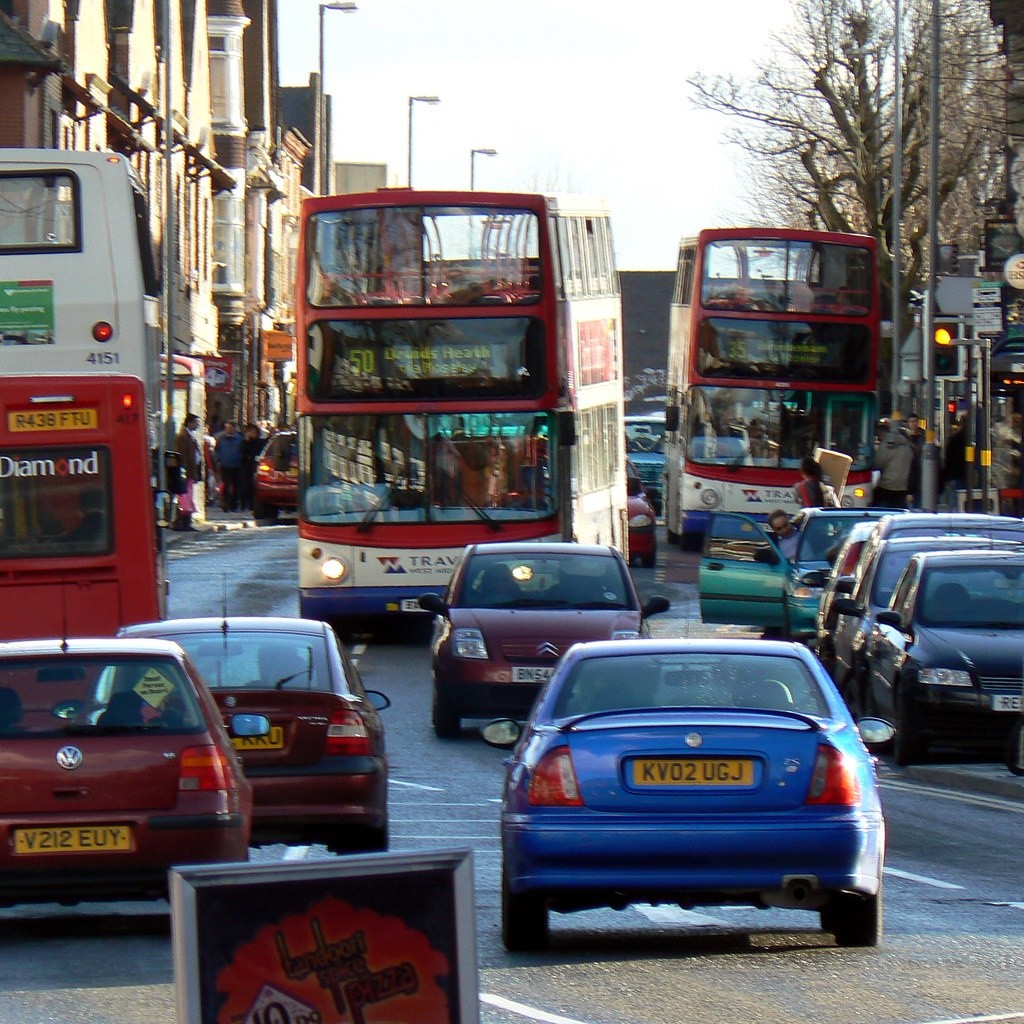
[932,320,960,375]
[947,400,956,425]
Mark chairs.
[468,288,550,304]
[0,687,27,736]
[247,645,306,685]
[733,680,821,712]
[706,289,767,311]
[98,693,150,728]
[358,288,419,305]
[470,564,524,606]
[553,571,610,603]
[571,680,656,713]
[932,583,982,621]
[804,296,867,317]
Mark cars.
[619,416,666,567]
[696,506,1024,775]
[0,615,395,907]
[253,430,301,519]
[480,636,898,954]
[418,542,672,739]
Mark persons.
[874,423,913,510]
[746,414,858,458]
[214,419,276,513]
[764,510,799,555]
[22,487,105,549]
[792,457,845,509]
[549,557,617,605]
[900,413,926,508]
[172,410,201,533]
[833,284,856,307]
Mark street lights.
[408,95,440,190]
[319,0,359,195]
[469,148,498,190]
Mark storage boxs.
[815,448,853,501]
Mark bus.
[293,187,631,623]
[0,146,171,648]
[662,226,883,550]
